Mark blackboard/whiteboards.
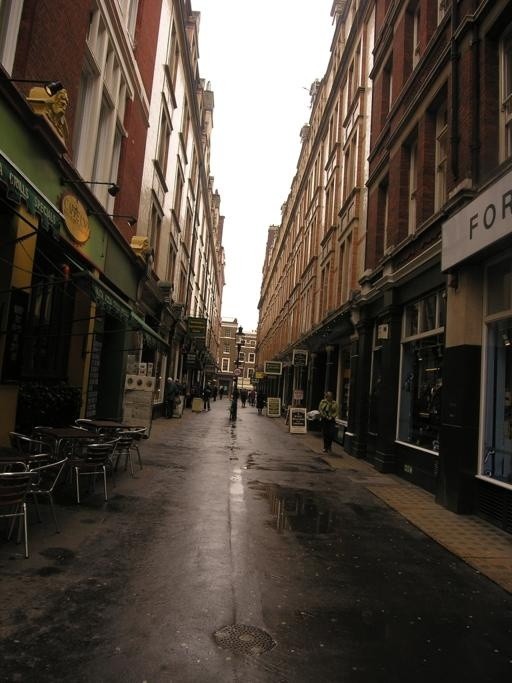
[267,398,281,418]
[290,408,307,434]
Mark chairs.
[0,415,148,560]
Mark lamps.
[60,177,138,227]
[9,78,62,97]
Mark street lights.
[229,325,246,421]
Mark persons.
[164,378,178,419]
[174,379,183,395]
[319,390,337,453]
[202,381,264,411]
